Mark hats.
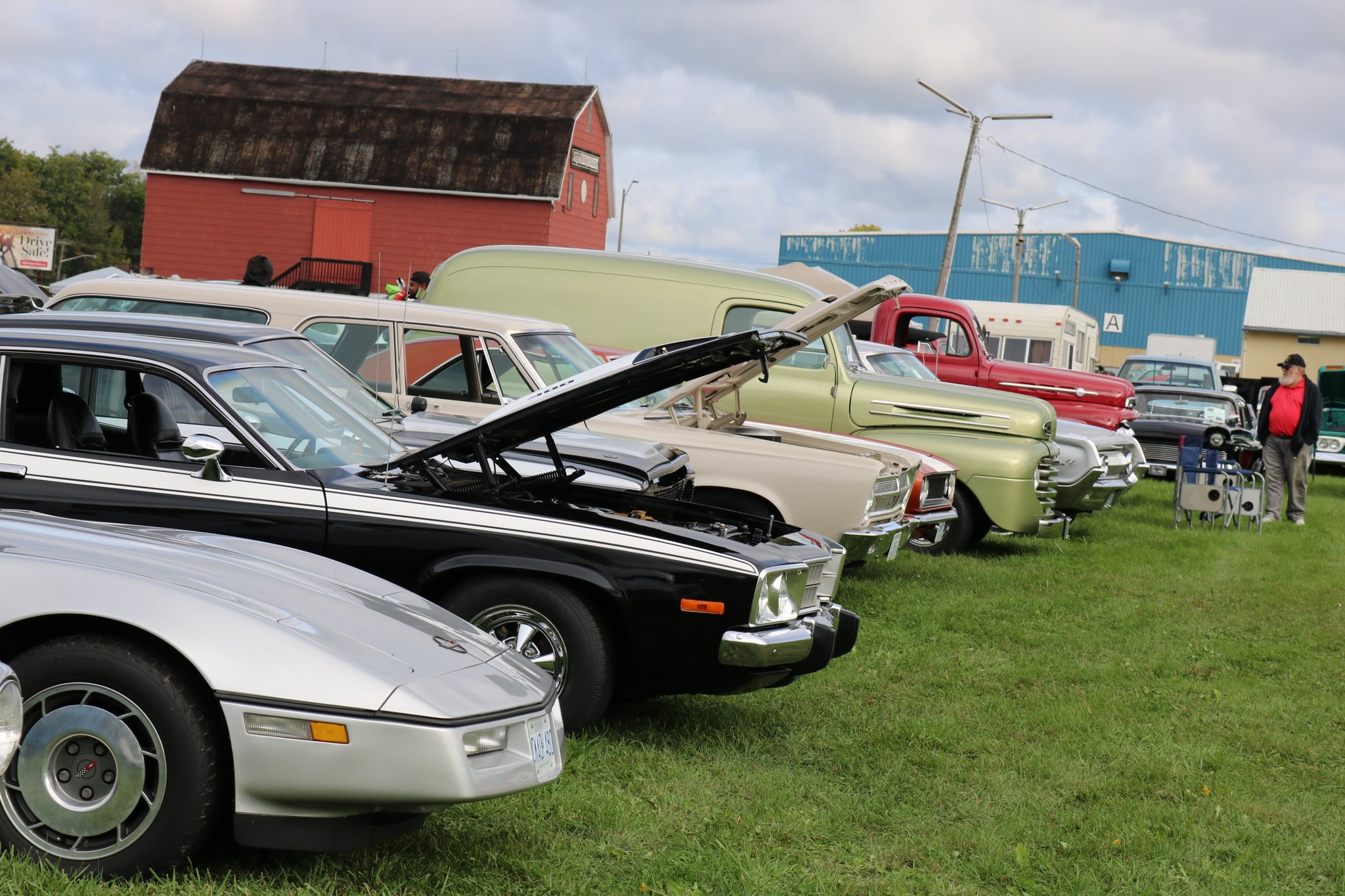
[1277,354,1306,368]
[243,255,273,286]
[411,271,430,285]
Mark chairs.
[128,392,191,462]
[1173,435,1265,534]
[142,373,203,424]
[46,392,106,452]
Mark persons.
[408,271,431,300]
[1256,354,1324,525]
[240,253,274,287]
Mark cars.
[1115,356,1345,477]
[751,329,1149,523]
[1,272,960,738]
[0,510,564,880]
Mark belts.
[1271,434,1293,439]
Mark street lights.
[979,196,1069,303]
[56,254,97,281]
[1060,232,1081,310]
[916,78,1053,330]
[618,180,639,251]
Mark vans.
[404,245,1070,559]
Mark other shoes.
[1261,516,1283,523]
[1293,519,1306,526]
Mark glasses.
[1281,365,1295,371]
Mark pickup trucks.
[870,293,1140,432]
